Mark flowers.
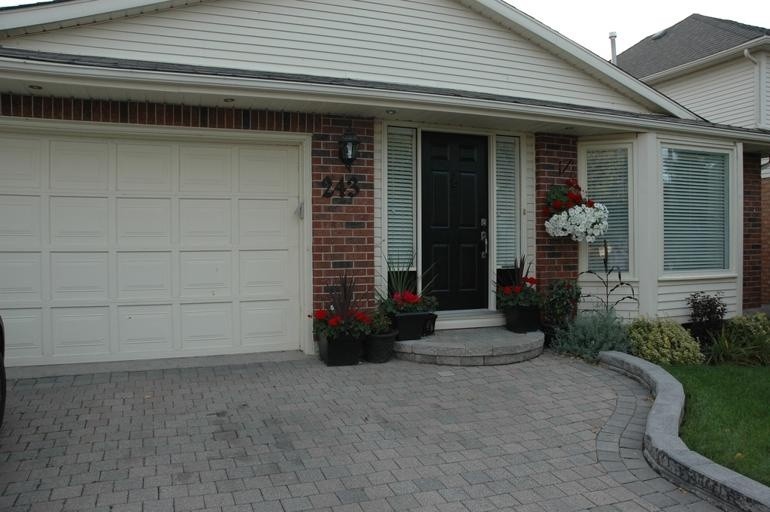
[489,254,546,308]
[311,246,441,341]
[542,182,609,242]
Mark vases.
[502,305,541,334]
[318,312,438,367]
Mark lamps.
[337,126,361,172]
